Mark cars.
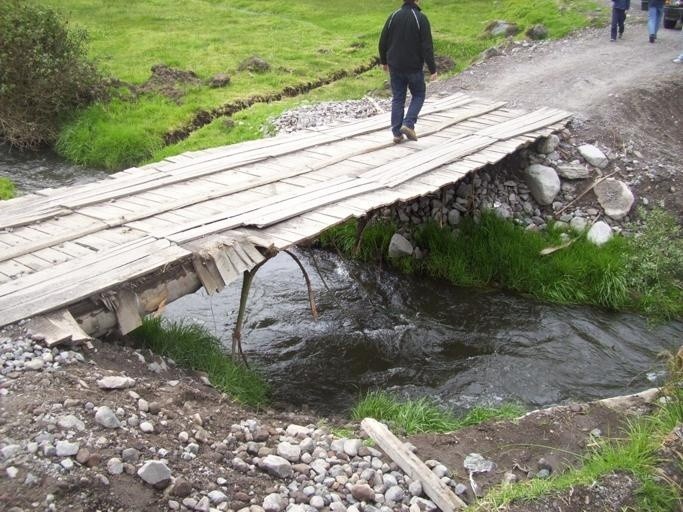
[663,1,683,29]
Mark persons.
[646,0,665,43]
[609,0,630,41]
[378,0,436,145]
[670,51,682,66]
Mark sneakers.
[393,134,403,142]
[399,126,416,140]
